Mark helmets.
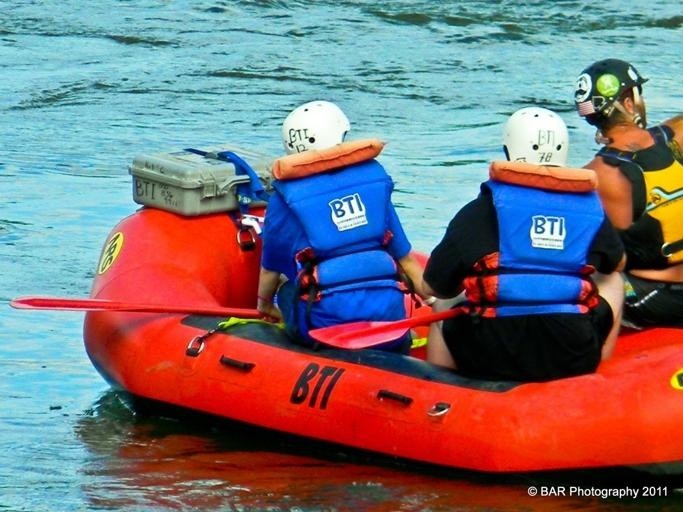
[281,100,350,156]
[575,59,649,124]
[502,107,569,167]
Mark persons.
[574,58,682,327]
[421,107,627,380]
[255,100,437,357]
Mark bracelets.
[258,296,273,304]
[422,295,437,306]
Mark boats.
[83,146,683,481]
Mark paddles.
[10,296,282,325]
[308,305,473,349]
